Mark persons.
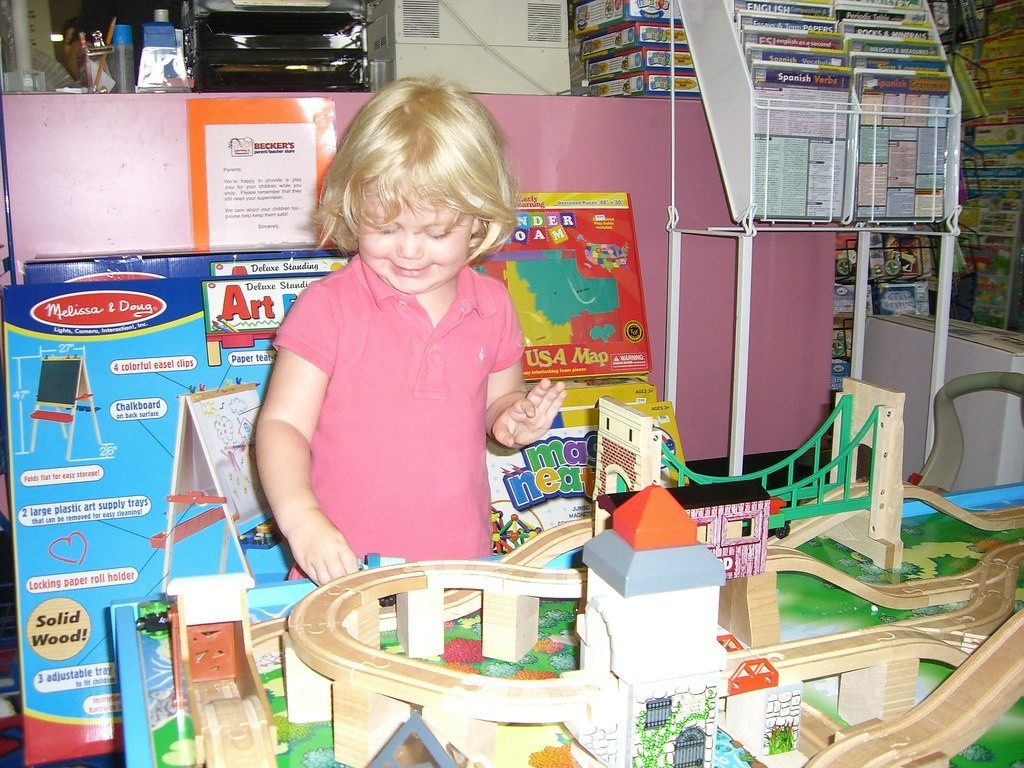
[253,72,570,591]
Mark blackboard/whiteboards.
[166,382,269,523]
[37,358,92,406]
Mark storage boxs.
[573,0,700,96]
